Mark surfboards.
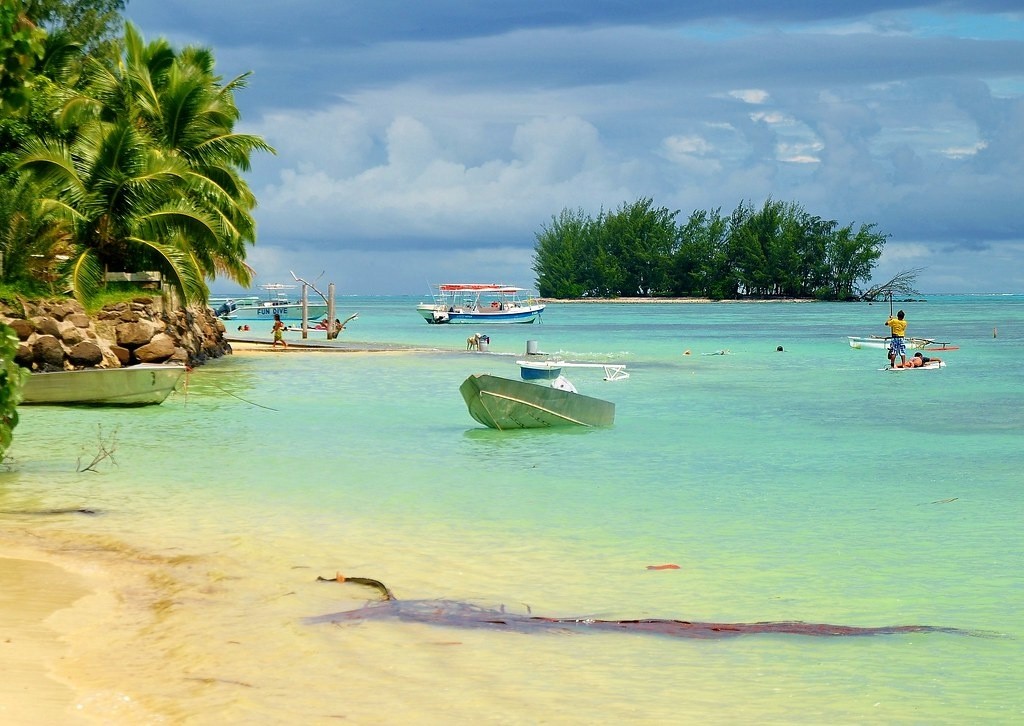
[876,360,946,371]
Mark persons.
[238,325,250,332]
[336,319,340,324]
[885,310,907,369]
[777,346,783,351]
[322,319,327,327]
[897,352,942,368]
[272,314,287,348]
[498,301,504,310]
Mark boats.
[416,279,546,325]
[206,270,358,341]
[847,334,935,350]
[17,359,188,407]
[519,366,563,378]
[458,373,616,431]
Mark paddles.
[887,290,894,359]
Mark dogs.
[466,333,481,351]
